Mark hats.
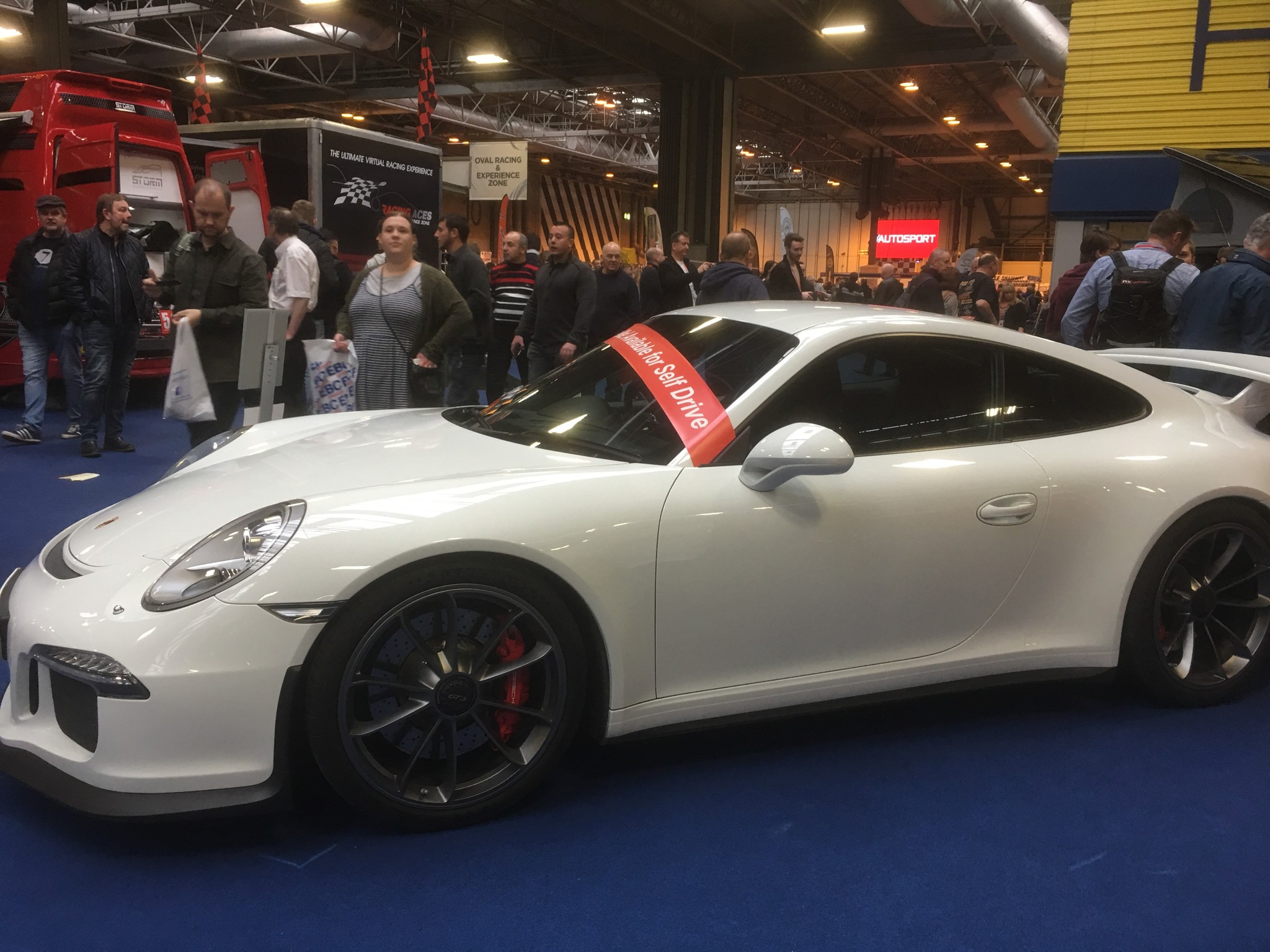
[35,194,66,208]
[942,266,960,282]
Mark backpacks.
[1020,293,1033,308]
[892,274,935,309]
[1092,250,1184,351]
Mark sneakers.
[1,427,42,443]
[61,424,81,439]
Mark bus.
[0,67,275,410]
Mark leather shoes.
[102,436,135,452]
[80,440,101,457]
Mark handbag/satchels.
[301,338,359,416]
[162,316,217,423]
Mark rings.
[429,365,431,368]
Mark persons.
[483,200,1270,440]
[365,217,410,267]
[257,199,354,341]
[60,192,156,459]
[439,214,495,411]
[140,178,270,449]
[0,193,84,446]
[328,213,473,412]
[261,205,321,418]
[318,228,344,257]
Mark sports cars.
[2,299,1270,832]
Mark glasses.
[1106,248,1120,252]
[1177,251,1191,257]
